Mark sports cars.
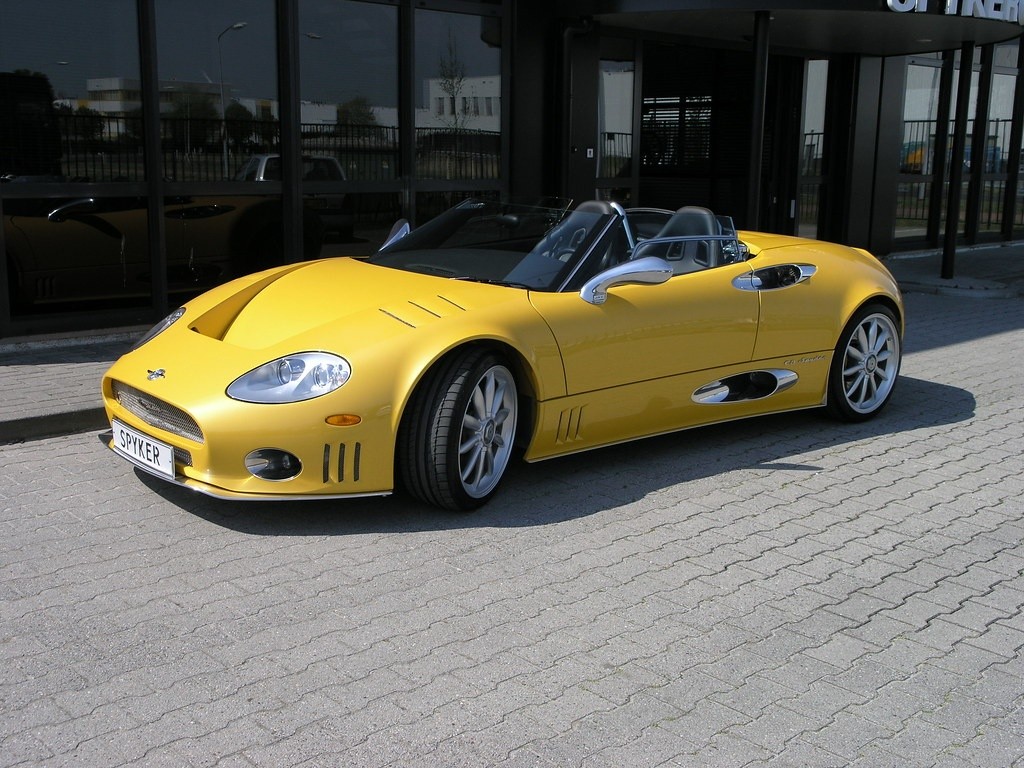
[98,198,905,513]
[0,172,267,293]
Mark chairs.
[630,206,721,275]
[536,202,621,272]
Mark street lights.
[33,59,69,76]
[218,20,248,179]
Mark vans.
[235,153,348,200]
